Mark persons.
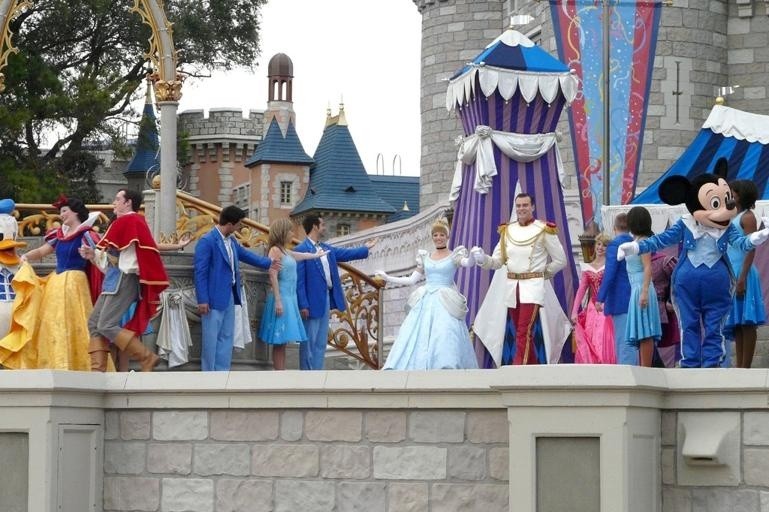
[619,205,664,367]
[572,232,616,365]
[258,219,335,370]
[290,212,372,371]
[192,204,281,372]
[715,177,766,369]
[0,191,118,373]
[475,189,566,366]
[78,188,164,372]
[598,210,641,365]
[372,220,478,371]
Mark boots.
[111,328,161,371]
[87,337,110,371]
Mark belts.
[508,273,543,279]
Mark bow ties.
[693,224,720,240]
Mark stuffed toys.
[613,157,769,368]
[0,198,31,371]
